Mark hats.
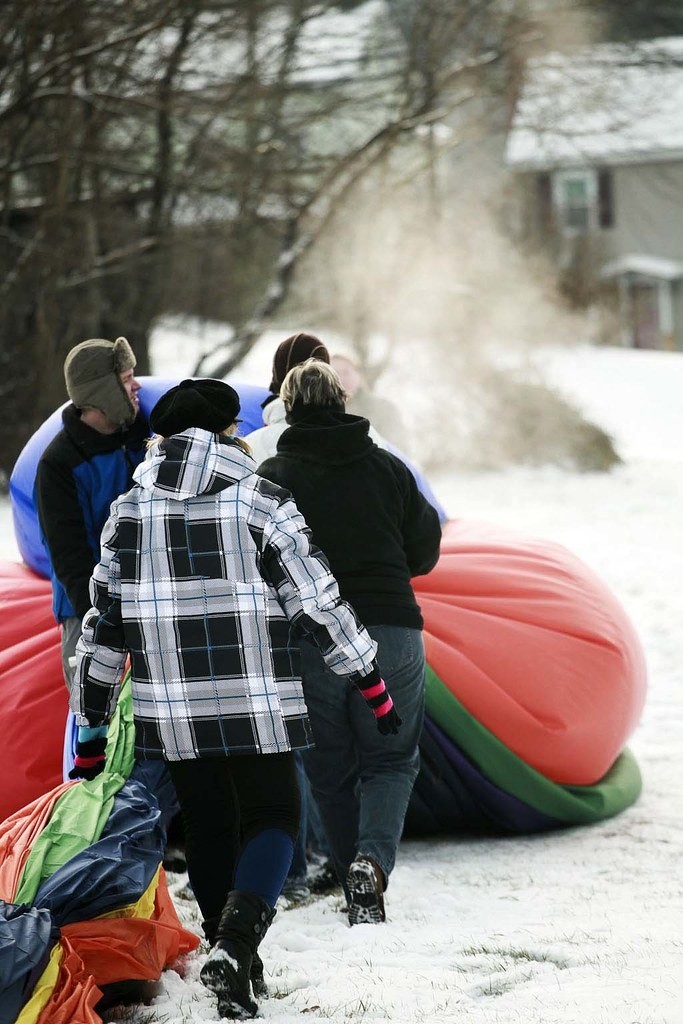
[273,333,330,387]
[150,379,240,438]
[63,336,137,426]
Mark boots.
[201,915,268,999]
[200,890,277,1020]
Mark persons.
[238,332,447,525]
[254,355,444,928]
[330,353,413,453]
[30,334,147,690]
[70,376,406,1022]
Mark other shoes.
[348,855,385,926]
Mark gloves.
[67,737,107,782]
[354,663,402,736]
